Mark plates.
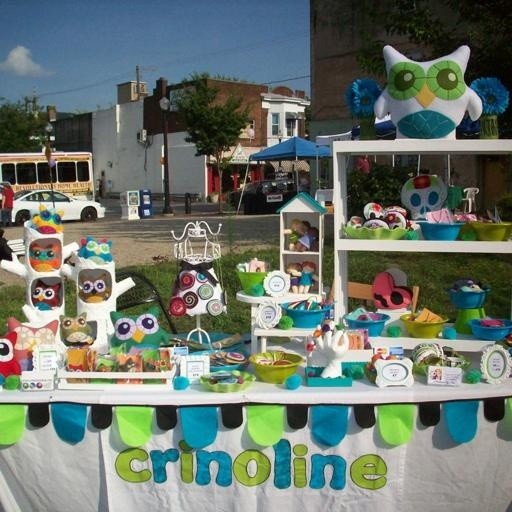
[200,370,256,393]
[343,225,408,240]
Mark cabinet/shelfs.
[332,139,512,354]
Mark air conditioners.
[136,130,146,143]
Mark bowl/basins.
[400,313,450,340]
[346,313,390,337]
[418,222,466,241]
[192,350,250,372]
[468,318,512,341]
[282,303,332,327]
[455,309,487,335]
[446,289,491,308]
[246,351,303,384]
[236,272,268,292]
[471,222,510,241]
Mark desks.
[1,371,512,511]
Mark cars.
[0,188,107,226]
[226,179,296,213]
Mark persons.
[285,217,318,251]
[2,182,16,229]
[304,322,353,387]
[285,257,319,294]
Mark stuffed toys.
[108,305,172,353]
[0,203,79,329]
[374,45,484,141]
[0,332,30,390]
[6,316,59,372]
[60,311,94,348]
[399,173,448,219]
[59,241,136,355]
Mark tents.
[235,135,330,215]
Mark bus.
[0,151,97,201]
